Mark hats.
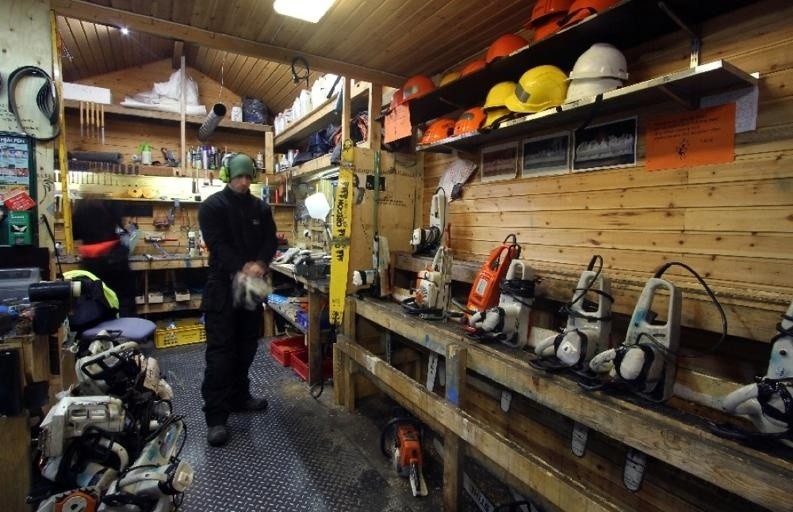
[229,154,255,178]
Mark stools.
[81,316,155,345]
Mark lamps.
[273,0,336,23]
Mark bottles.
[186,143,229,170]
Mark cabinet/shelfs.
[381,0,759,153]
[55,91,274,329]
[265,74,413,182]
[267,248,335,386]
[341,265,793,512]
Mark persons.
[196,150,278,447]
[70,173,139,319]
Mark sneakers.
[206,424,228,443]
[230,395,267,412]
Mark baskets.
[270,336,333,381]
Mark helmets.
[388,0,625,144]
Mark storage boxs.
[270,331,306,367]
[290,347,309,382]
